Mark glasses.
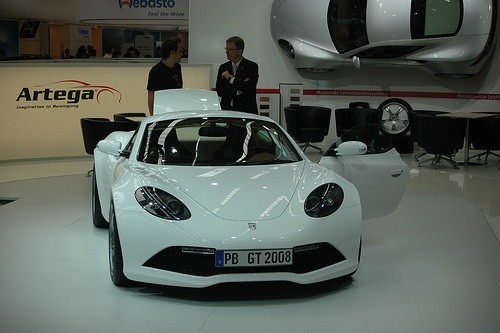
[224,47,238,50]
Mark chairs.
[80,98,500,178]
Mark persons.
[146,36,185,117]
[78,46,89,59]
[63,48,76,59]
[123,46,140,58]
[216,35,260,115]
[87,44,98,57]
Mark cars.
[89,87,365,296]
[270,0,497,81]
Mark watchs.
[227,74,234,82]
